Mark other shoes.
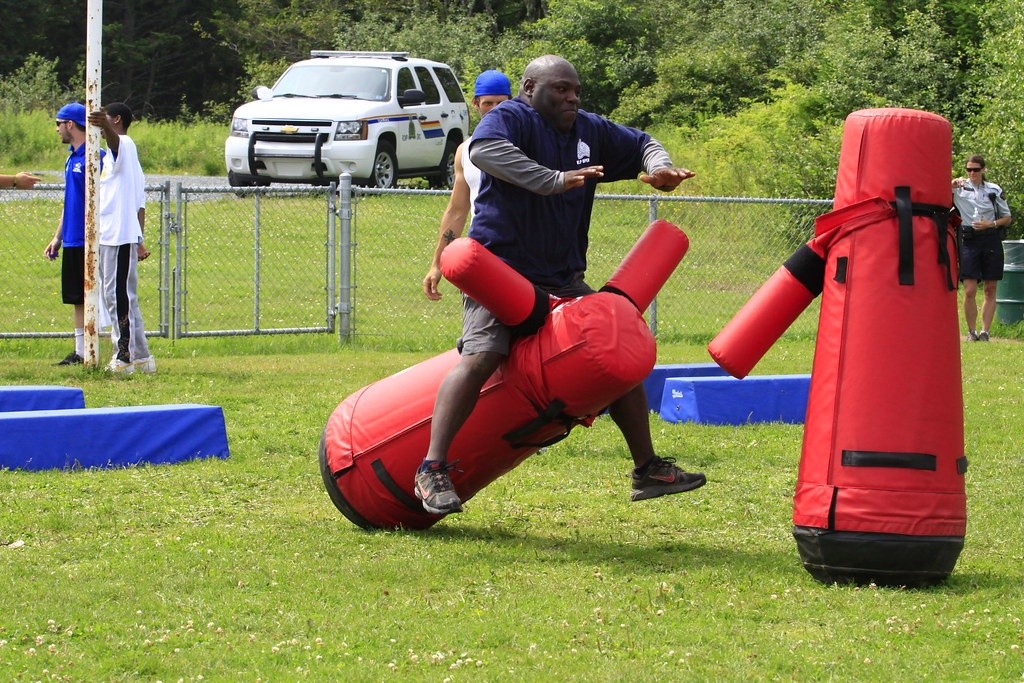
[978,332,989,341]
[966,333,978,341]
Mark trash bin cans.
[996,241,1024,323]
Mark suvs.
[221,50,470,192]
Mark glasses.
[56,120,69,126]
[966,167,982,172]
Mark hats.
[55,102,85,125]
[475,69,511,95]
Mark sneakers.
[58,351,84,365]
[105,361,134,373]
[632,456,706,500]
[134,355,156,373]
[414,460,466,513]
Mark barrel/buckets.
[995,241,1024,326]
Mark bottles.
[973,207,981,221]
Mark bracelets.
[994,220,997,228]
[13,174,17,189]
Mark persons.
[88,102,156,375]
[417,56,707,513]
[951,155,1011,342]
[423,70,512,300]
[0,172,42,189]
[43,102,106,365]
